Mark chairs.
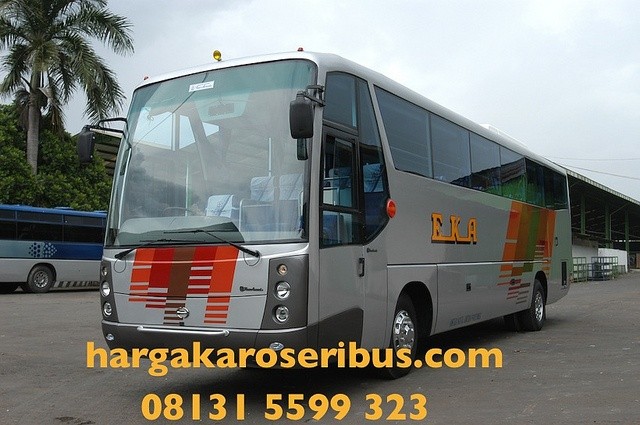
[249,176,277,220]
[328,168,352,190]
[277,174,303,226]
[361,162,384,241]
[204,194,238,220]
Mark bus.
[98,47,574,382]
[0,203,106,294]
